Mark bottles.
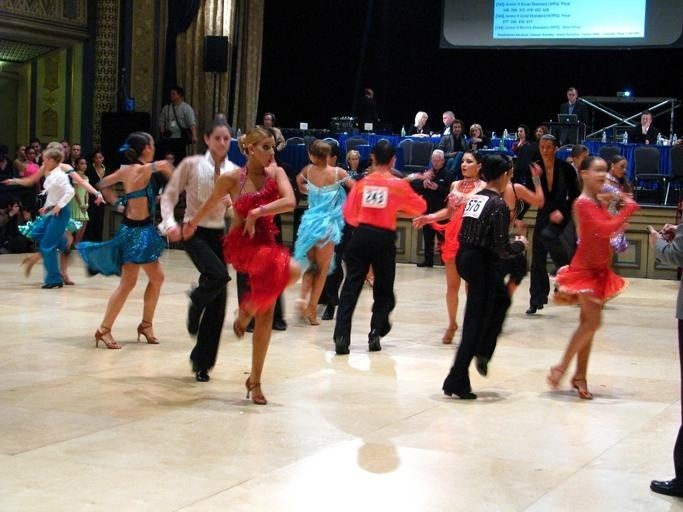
[601,132,607,143]
[672,134,677,145]
[623,131,628,144]
[401,127,405,137]
[492,132,497,139]
[499,137,504,151]
[657,132,662,145]
[237,128,241,140]
[503,129,508,140]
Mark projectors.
[616,91,631,96]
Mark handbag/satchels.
[181,128,194,144]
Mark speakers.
[203,35,228,72]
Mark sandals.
[443,324,457,343]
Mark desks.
[227,122,683,188]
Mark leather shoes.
[21,255,74,288]
[444,390,476,398]
[474,354,487,376]
[194,360,209,382]
[188,303,199,333]
[234,303,382,354]
[525,305,543,313]
[651,481,682,497]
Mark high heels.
[571,376,592,399]
[95,325,121,348]
[138,320,158,344]
[246,377,267,406]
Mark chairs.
[664,145,683,205]
[634,146,665,203]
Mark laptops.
[558,113,578,124]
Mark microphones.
[121,67,126,88]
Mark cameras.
[164,129,172,137]
[18,200,33,212]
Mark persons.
[644,222,683,501]
[1,85,658,405]
